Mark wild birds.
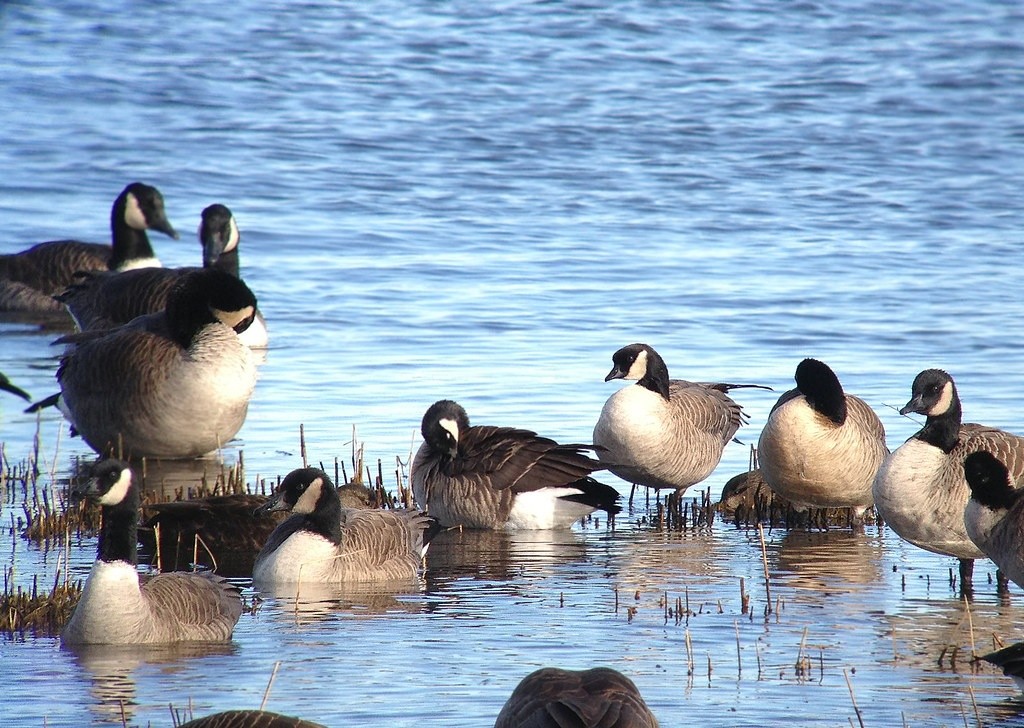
[1,183,1022,728]
